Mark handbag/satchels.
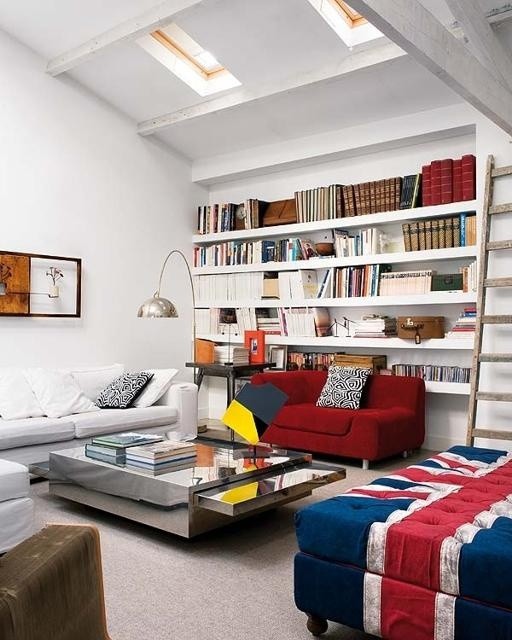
[396,315,446,346]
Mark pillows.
[28,368,97,418]
[97,370,152,408]
[317,365,371,410]
[131,367,177,407]
[0,368,42,419]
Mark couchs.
[0,365,199,555]
[290,443,512,635]
[0,524,110,640]
[250,369,427,470]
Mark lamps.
[132,249,199,384]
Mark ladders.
[466,154,512,447]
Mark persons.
[339,270,341,298]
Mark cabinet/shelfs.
[189,199,477,455]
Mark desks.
[184,361,277,441]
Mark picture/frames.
[0,250,29,317]
[29,255,83,319]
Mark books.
[402,223,412,252]
[355,314,397,338]
[334,228,394,258]
[125,451,197,464]
[288,350,346,371]
[334,268,336,298]
[432,220,438,249]
[453,160,463,202]
[444,308,477,340]
[198,199,268,235]
[313,308,334,337]
[460,213,465,247]
[331,268,333,297]
[341,177,396,216]
[462,155,476,201]
[195,238,314,267]
[445,217,453,248]
[214,345,249,363]
[465,217,472,247]
[342,261,480,297]
[317,270,330,299]
[453,217,460,247]
[424,221,428,249]
[422,165,431,207]
[92,432,164,448]
[418,220,426,251]
[380,364,471,383]
[295,185,346,223]
[472,216,476,246]
[193,270,319,299]
[410,222,418,251]
[86,443,125,456]
[321,271,331,298]
[397,173,422,211]
[126,440,197,459]
[195,307,316,336]
[438,219,445,249]
[431,160,440,205]
[336,269,340,298]
[441,159,453,204]
